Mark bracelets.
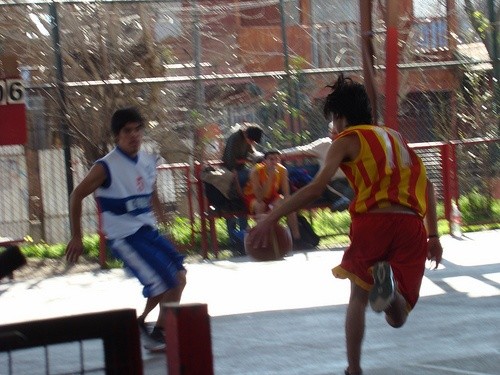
[427,234,439,238]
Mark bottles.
[450,204,462,236]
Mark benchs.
[187,149,439,259]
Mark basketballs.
[244,221,293,262]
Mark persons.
[222,123,263,198]
[64,107,187,353]
[248,74,444,374]
[249,148,315,250]
